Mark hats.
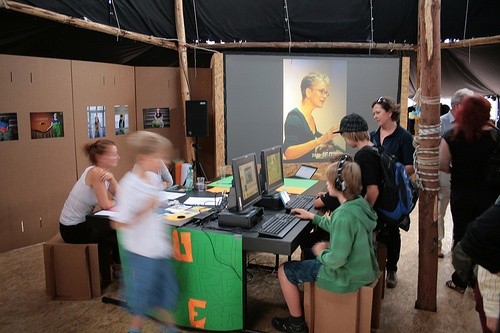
[332,112,368,134]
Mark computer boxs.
[218,206,264,228]
[253,191,285,210]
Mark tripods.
[182,137,208,187]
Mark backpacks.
[361,143,419,216]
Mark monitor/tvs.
[231,152,262,212]
[260,145,285,193]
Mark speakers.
[185,100,208,137]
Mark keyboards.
[285,195,315,214]
[258,213,301,239]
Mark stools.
[43,231,111,299]
[304,272,384,333]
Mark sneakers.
[271,316,309,333]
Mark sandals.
[446,280,465,294]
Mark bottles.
[186,167,194,189]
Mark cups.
[197,177,206,192]
[220,167,227,180]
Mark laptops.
[285,164,318,179]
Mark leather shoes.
[386,271,398,287]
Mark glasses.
[310,87,330,97]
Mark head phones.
[335,156,348,191]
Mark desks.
[98,174,329,332]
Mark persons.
[331,113,397,248]
[404,101,451,137]
[366,96,421,288]
[93,116,102,137]
[452,189,500,333]
[299,151,355,261]
[152,108,165,127]
[281,72,345,164]
[47,113,63,137]
[118,114,126,135]
[109,130,186,333]
[436,86,477,257]
[271,161,380,333]
[436,92,499,294]
[59,138,124,296]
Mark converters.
[193,211,214,225]
